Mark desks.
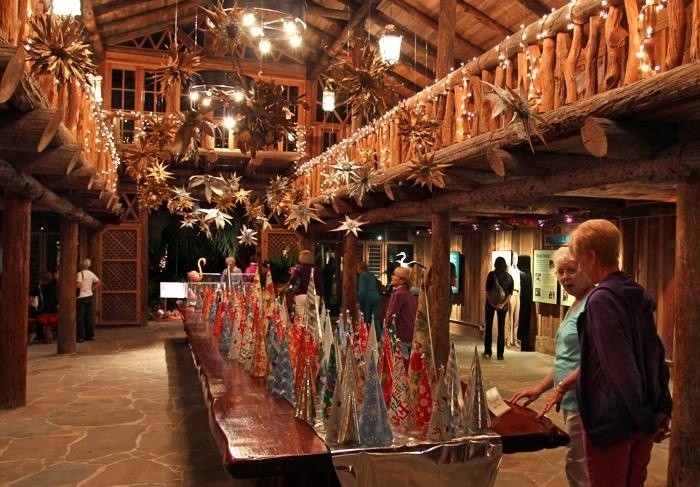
[177,302,571,487]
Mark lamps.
[205,0,307,58]
[319,39,336,112]
[189,69,258,107]
[379,0,403,66]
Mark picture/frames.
[450,251,459,295]
[492,249,512,272]
[533,249,559,306]
[559,285,576,306]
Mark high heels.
[481,350,492,358]
[497,355,504,360]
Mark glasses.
[553,266,583,279]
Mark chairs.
[28,282,58,344]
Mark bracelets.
[539,381,551,390]
[557,381,568,394]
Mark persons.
[33,272,58,339]
[480,256,514,360]
[76,258,102,343]
[567,218,674,486]
[384,266,418,359]
[424,262,457,319]
[356,262,382,342]
[218,249,335,302]
[510,244,591,487]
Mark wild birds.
[395,252,428,269]
[187,257,206,282]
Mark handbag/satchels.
[489,277,506,305]
[76,287,80,297]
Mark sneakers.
[77,337,95,343]
